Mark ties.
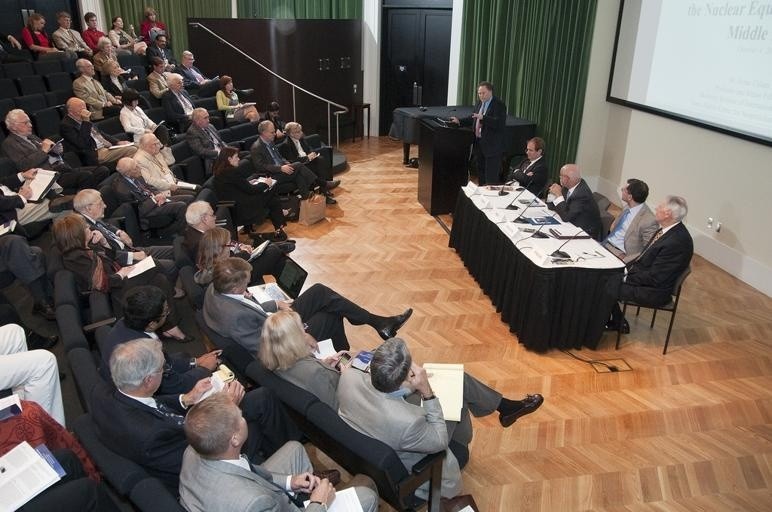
[241,453,306,509]
[96,222,116,240]
[161,50,166,59]
[26,136,42,149]
[634,229,663,262]
[134,179,152,197]
[91,79,104,97]
[474,101,485,139]
[177,91,192,110]
[599,208,630,245]
[67,30,83,48]
[160,72,168,80]
[266,145,284,165]
[565,191,571,204]
[189,67,205,82]
[154,396,185,425]
[204,129,215,143]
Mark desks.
[449,181,626,352]
[470,115,537,155]
[390,105,478,164]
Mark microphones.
[444,109,456,126]
[513,191,544,223]
[531,205,564,238]
[499,173,513,196]
[551,225,593,258]
[506,183,529,210]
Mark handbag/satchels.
[298,195,327,226]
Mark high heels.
[158,329,196,343]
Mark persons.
[604,192,694,334]
[600,179,660,265]
[547,164,602,242]
[1,6,545,512]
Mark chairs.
[592,192,615,241]
[615,265,692,355]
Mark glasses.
[221,240,238,248]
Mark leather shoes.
[270,241,295,254]
[172,287,188,300]
[498,393,543,428]
[322,179,341,191]
[605,318,631,335]
[325,197,336,205]
[29,332,59,349]
[313,469,340,486]
[379,307,414,341]
[32,304,56,320]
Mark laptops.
[246,256,308,305]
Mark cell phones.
[335,353,352,371]
